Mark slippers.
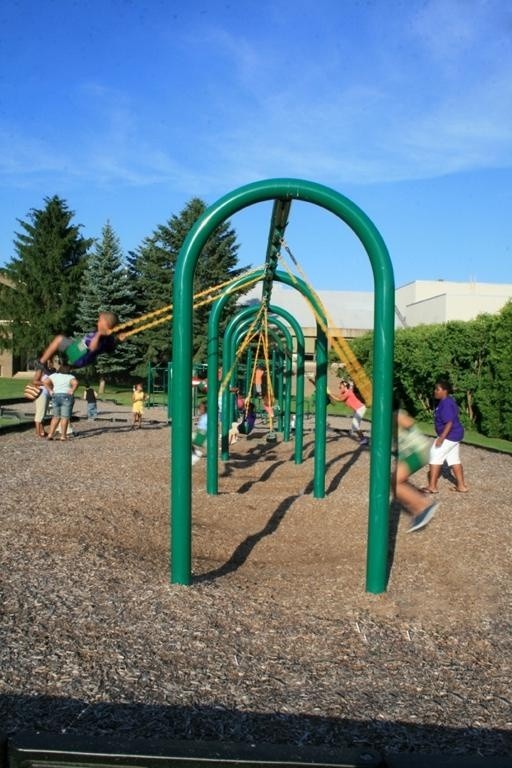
[450,486,468,492]
[419,487,439,492]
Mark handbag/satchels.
[24,383,41,400]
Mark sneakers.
[404,498,442,534]
[28,358,46,371]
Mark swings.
[273,239,431,474]
[58,263,266,369]
[261,317,367,420]
[237,330,260,435]
[260,308,279,443]
[192,304,265,448]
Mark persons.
[327,381,370,444]
[423,378,470,493]
[33,311,119,376]
[218,384,256,444]
[130,383,148,429]
[32,348,78,440]
[192,401,208,457]
[84,383,98,421]
[386,390,441,536]
